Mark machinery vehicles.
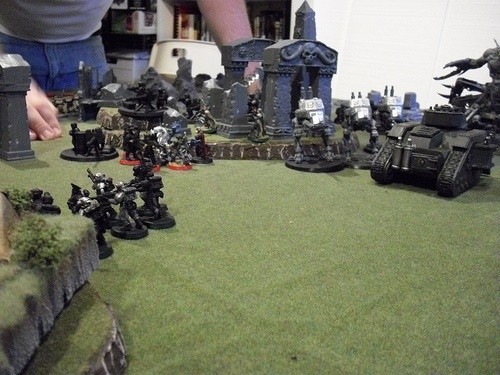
[370,102,494,199]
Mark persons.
[0,0,260,144]
[25,78,216,255]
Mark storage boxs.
[112,9,157,34]
[106,50,152,86]
